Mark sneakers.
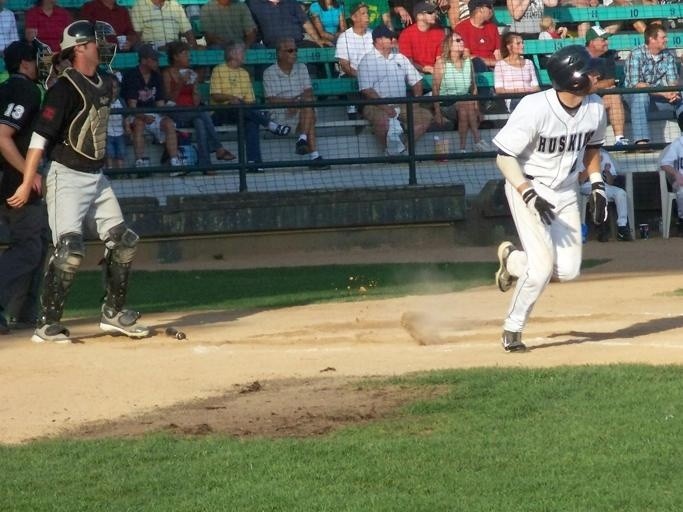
[501,329,526,354]
[308,158,330,170]
[614,135,633,151]
[107,149,237,176]
[30,321,72,345]
[99,304,150,338]
[616,227,632,240]
[495,240,517,292]
[597,232,609,242]
[296,139,308,155]
[473,140,497,152]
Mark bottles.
[346,106,357,120]
[581,223,586,243]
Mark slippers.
[268,124,291,136]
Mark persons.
[658,112,683,237]
[8,18,151,345]
[491,44,609,353]
[0,1,683,179]
[578,147,632,242]
[0,38,52,335]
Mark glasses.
[280,47,298,53]
[452,37,465,41]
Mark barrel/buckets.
[434,137,449,154]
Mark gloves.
[588,180,609,224]
[521,188,556,226]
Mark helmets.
[60,19,95,48]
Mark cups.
[638,224,649,240]
[115,36,126,50]
[433,135,448,163]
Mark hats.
[586,25,612,44]
[547,44,618,93]
[136,45,161,61]
[373,26,396,41]
[414,3,436,17]
[350,2,369,14]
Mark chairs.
[578,155,636,240]
[656,142,683,240]
